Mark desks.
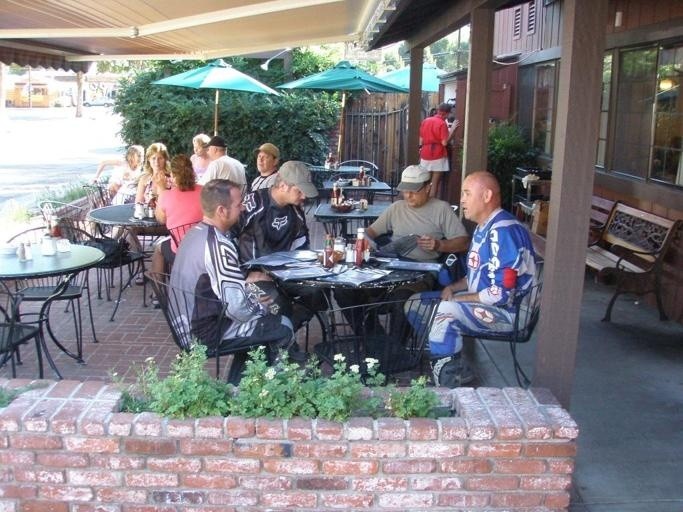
[260,256,440,366]
[0,241,108,363]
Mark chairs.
[31,159,464,321]
[451,280,547,388]
[1,225,98,366]
[142,269,277,384]
[0,289,50,380]
[308,295,445,386]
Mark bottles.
[18,240,31,262]
[322,227,369,269]
[350,165,371,186]
[330,182,366,211]
[147,207,153,218]
[43,218,60,237]
[323,152,339,169]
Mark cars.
[82,96,115,108]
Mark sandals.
[148,293,161,309]
[134,269,145,285]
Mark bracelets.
[434,240,440,251]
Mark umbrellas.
[150,59,279,137]
[277,61,408,168]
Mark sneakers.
[287,340,308,362]
[456,360,475,384]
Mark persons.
[197,136,247,194]
[190,133,211,178]
[404,170,538,384]
[166,178,295,369]
[419,102,460,197]
[149,155,204,308]
[226,160,319,362]
[108,143,172,285]
[332,164,470,370]
[251,143,280,191]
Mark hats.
[279,159,320,199]
[254,142,281,158]
[438,103,453,113]
[202,136,228,148]
[395,163,432,192]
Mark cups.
[58,238,71,252]
[39,237,56,255]
[132,202,146,219]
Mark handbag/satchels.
[85,222,132,267]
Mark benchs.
[585,201,683,323]
[532,193,618,280]
